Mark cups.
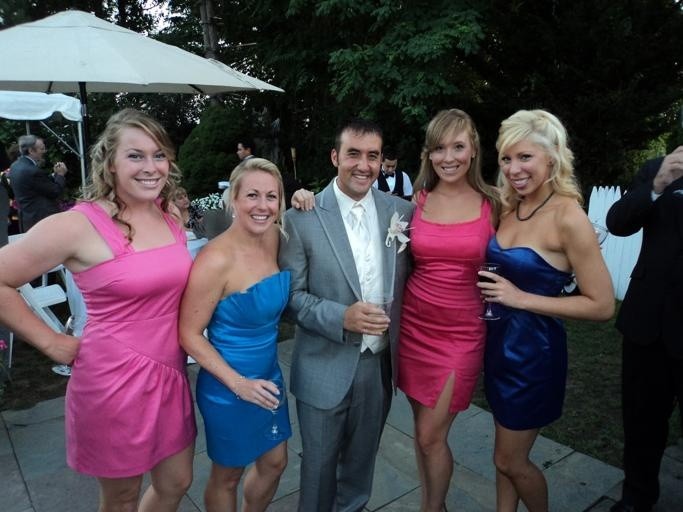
[365,292,395,334]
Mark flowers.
[385,211,416,254]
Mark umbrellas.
[0,7,286,200]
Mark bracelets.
[234,376,246,399]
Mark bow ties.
[385,173,394,178]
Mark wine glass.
[478,262,504,322]
[51,314,86,377]
[262,379,288,441]
[591,221,608,245]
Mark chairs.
[8,282,67,369]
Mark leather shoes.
[611,492,659,511]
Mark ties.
[350,206,388,355]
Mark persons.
[178,157,315,512]
[10,135,68,234]
[173,187,203,229]
[282,118,417,512]
[0,108,193,512]
[372,153,414,202]
[236,139,255,160]
[394,108,498,512]
[476,109,616,511]
[606,125,682,511]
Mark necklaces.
[516,189,556,221]
